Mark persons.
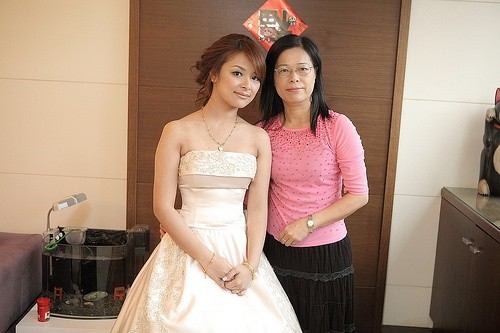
[159,34,370,333]
[110,33,303,333]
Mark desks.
[15,299,117,333]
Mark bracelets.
[204,252,215,273]
[244,262,256,280]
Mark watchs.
[307,214,314,233]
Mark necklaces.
[201,106,238,151]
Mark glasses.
[273,64,317,77]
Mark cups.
[37,297,50,322]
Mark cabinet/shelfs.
[429,186,500,333]
